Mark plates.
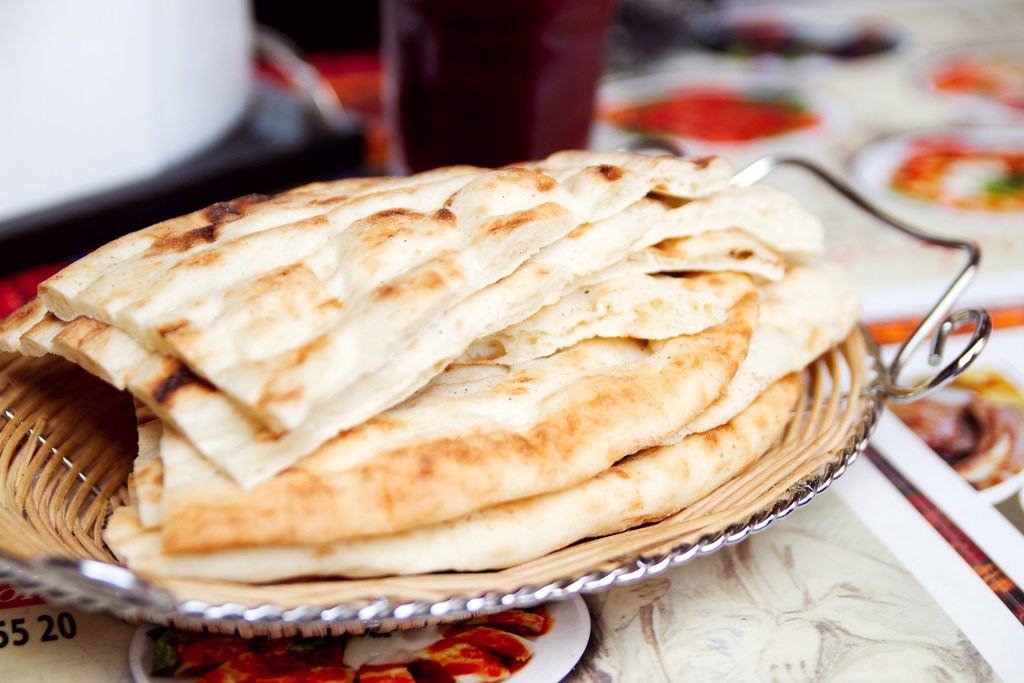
[908,39,1024,130]
[848,124,1024,244]
[686,10,902,62]
[595,76,845,155]
[891,389,1023,506]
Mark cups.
[382,0,614,179]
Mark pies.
[1,137,853,584]
